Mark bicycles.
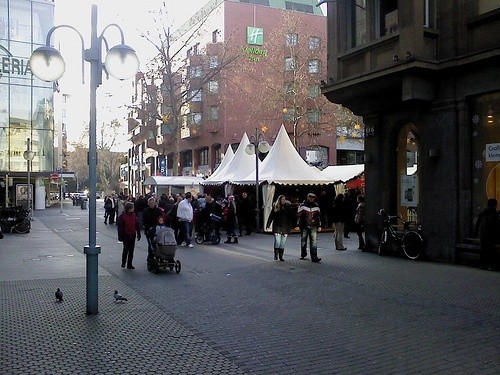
[376,209,425,261]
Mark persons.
[333,194,347,251]
[266,194,297,261]
[117,202,141,269]
[355,195,367,249]
[149,217,166,244]
[104,191,253,247]
[333,192,350,238]
[142,197,166,269]
[297,193,321,263]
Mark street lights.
[245,126,270,234]
[27,2,141,315]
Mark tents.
[199,124,365,232]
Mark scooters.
[194,221,221,245]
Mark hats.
[308,193,317,198]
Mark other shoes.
[121,263,125,268]
[339,247,347,250]
[179,244,184,246]
[188,244,194,247]
[300,257,306,260]
[104,221,107,225]
[127,265,135,269]
[313,258,321,261]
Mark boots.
[224,236,231,243]
[274,247,279,260]
[231,236,238,244]
[279,248,284,261]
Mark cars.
[86,192,100,199]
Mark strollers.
[145,223,181,274]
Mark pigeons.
[54,287,64,302]
[112,289,128,304]
